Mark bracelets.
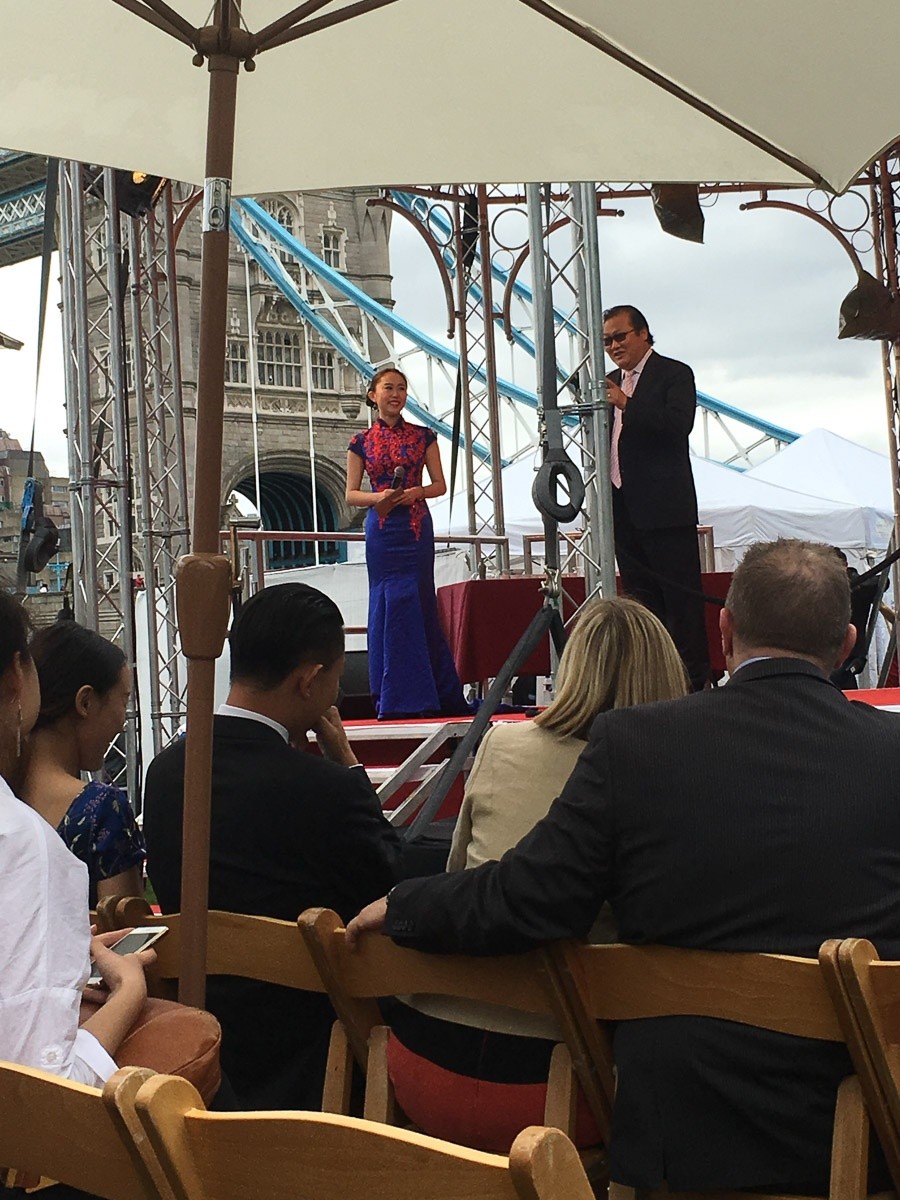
[418,486,425,501]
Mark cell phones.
[85,925,169,989]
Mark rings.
[608,393,611,398]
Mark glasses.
[603,330,634,347]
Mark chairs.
[0,908,900,1200]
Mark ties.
[610,373,633,490]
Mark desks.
[437,572,733,712]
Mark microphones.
[390,466,405,489]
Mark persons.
[345,368,477,722]
[344,535,900,1199]
[386,597,692,1157]
[1,583,158,1087]
[141,581,422,1115]
[585,304,714,689]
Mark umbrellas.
[0,3,900,1005]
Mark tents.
[369,421,899,688]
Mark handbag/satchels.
[77,989,224,1111]
